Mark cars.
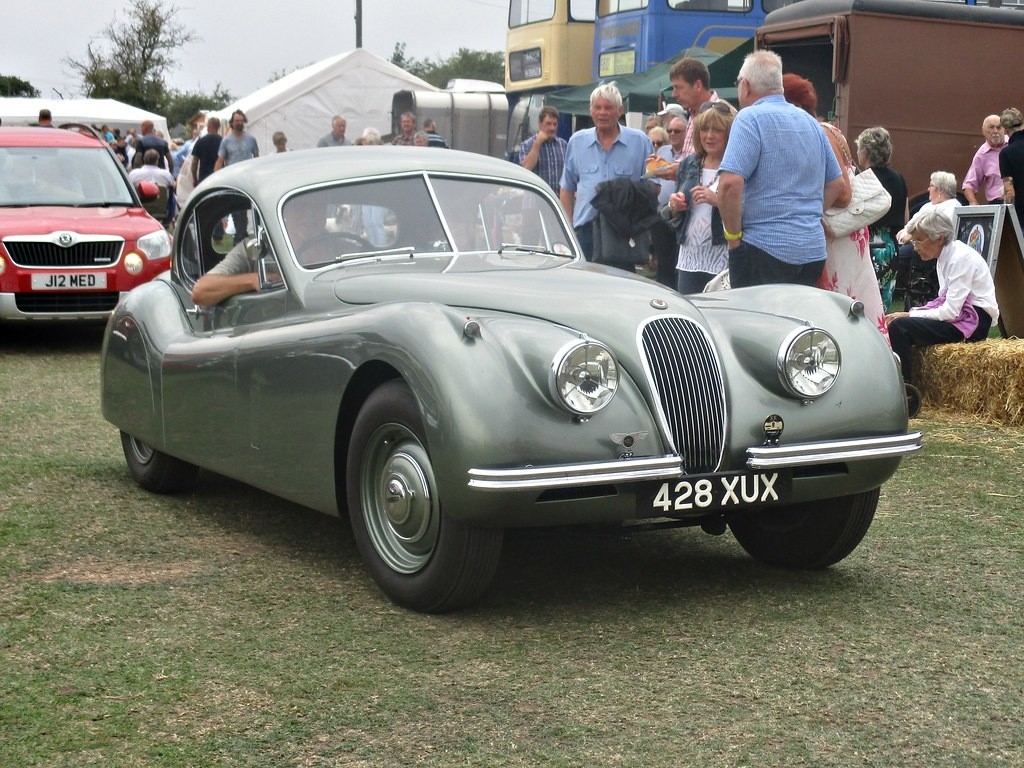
[0,122,174,323]
[99,146,925,614]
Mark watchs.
[723,228,743,240]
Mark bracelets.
[668,201,672,211]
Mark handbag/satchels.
[591,211,650,265]
[820,124,892,237]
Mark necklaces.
[698,152,724,188]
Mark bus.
[504,0,593,97]
[591,0,805,90]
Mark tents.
[197,42,442,234]
[0,97,174,153]
[540,44,743,140]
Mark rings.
[701,194,703,198]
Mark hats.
[656,104,685,117]
[1000,107,1023,129]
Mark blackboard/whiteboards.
[952,204,1024,340]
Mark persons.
[391,112,450,149]
[961,111,1010,204]
[190,189,339,307]
[317,114,384,148]
[715,49,846,289]
[884,209,1000,395]
[853,126,910,314]
[518,109,569,245]
[895,170,963,291]
[556,80,655,273]
[659,102,736,294]
[37,107,293,247]
[646,62,738,288]
[998,106,1024,229]
[783,73,893,350]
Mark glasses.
[734,78,750,88]
[666,128,686,134]
[651,141,665,148]
[699,102,733,117]
[909,238,930,249]
